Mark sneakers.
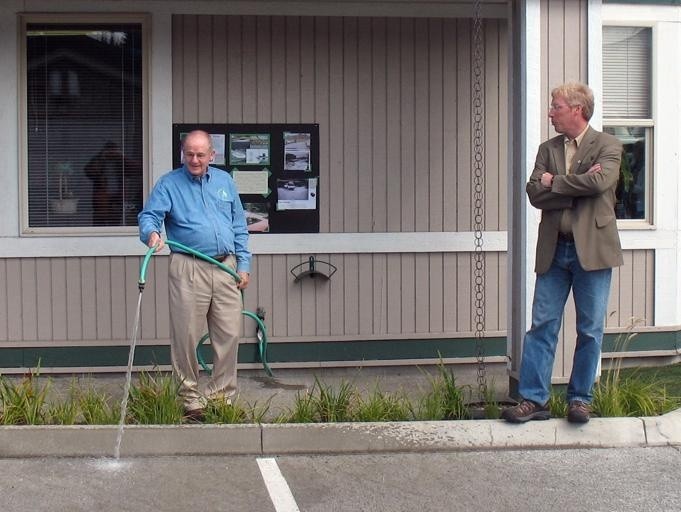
[182,405,248,423]
[567,400,590,422]
[501,395,551,423]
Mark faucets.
[257,307,266,324]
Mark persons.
[502,84,625,423]
[628,140,647,219]
[137,130,253,424]
[84,137,141,227]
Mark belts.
[174,250,230,265]
[555,231,579,242]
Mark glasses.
[548,104,579,111]
